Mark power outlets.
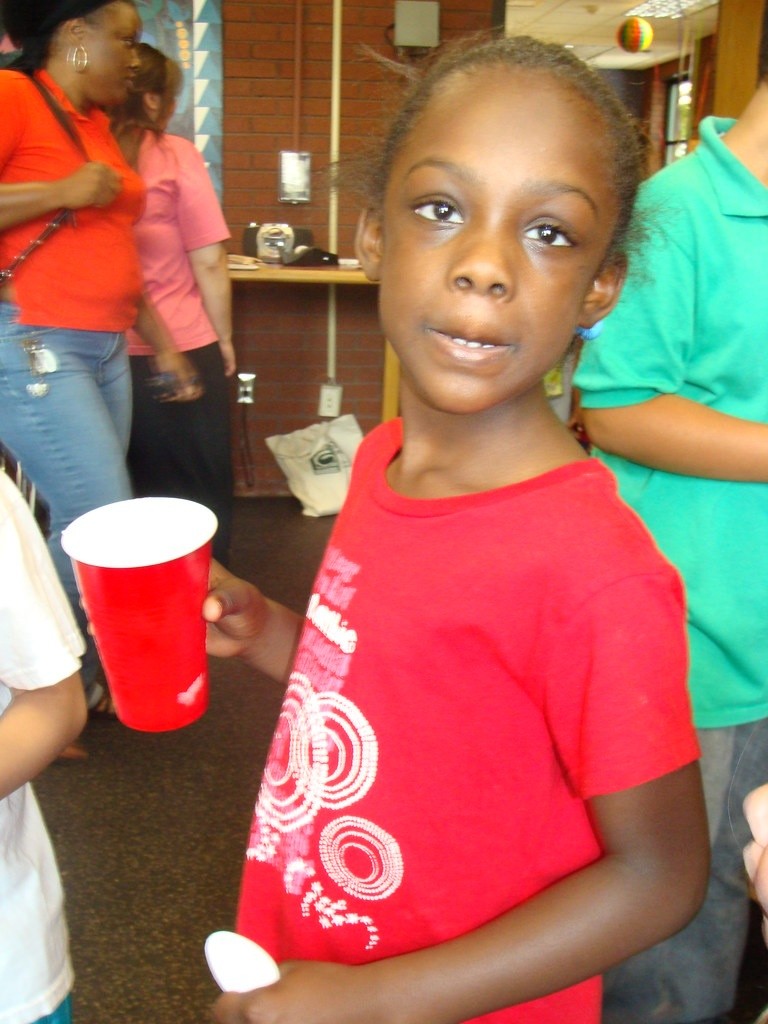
[318,385,343,416]
[237,373,256,404]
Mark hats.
[0,0,108,38]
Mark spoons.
[201,931,282,997]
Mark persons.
[0,1,236,758]
[81,36,709,1024]
[571,0,768,1024]
[0,467,89,1024]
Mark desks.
[227,262,400,422]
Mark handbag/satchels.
[266,414,364,518]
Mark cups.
[59,496,217,732]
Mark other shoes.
[88,681,115,721]
